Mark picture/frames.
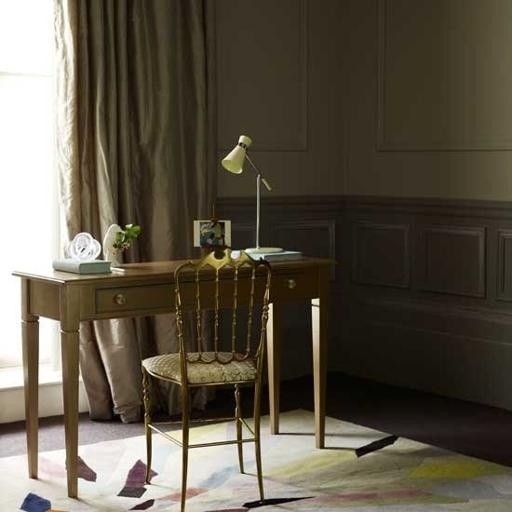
[193,218,231,248]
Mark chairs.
[141,248,274,512]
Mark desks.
[10,254,337,501]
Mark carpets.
[1,408,511,512]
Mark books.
[52,258,111,274]
[229,248,304,263]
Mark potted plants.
[103,222,143,267]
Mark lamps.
[220,133,284,255]
